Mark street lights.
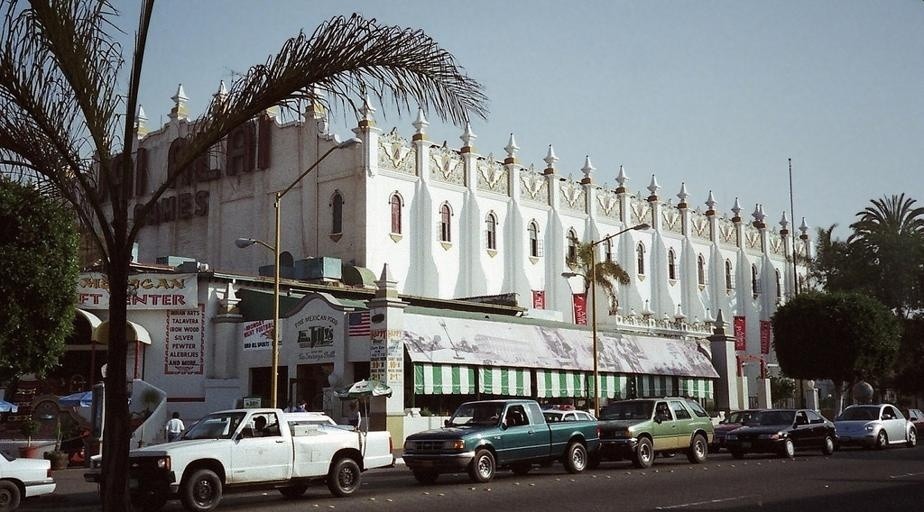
[562,222,650,418]
[235,136,363,408]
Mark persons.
[251,415,270,437]
[345,400,361,428]
[282,401,297,412]
[294,399,307,412]
[163,409,184,443]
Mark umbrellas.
[332,377,391,432]
[57,390,93,409]
[0,397,18,413]
